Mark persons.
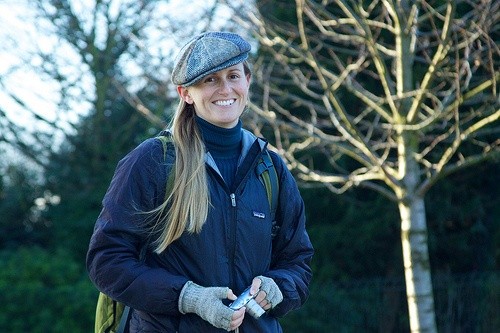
[85,30,317,333]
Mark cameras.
[228,284,266,320]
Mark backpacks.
[93,135,281,333]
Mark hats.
[170,30,251,87]
